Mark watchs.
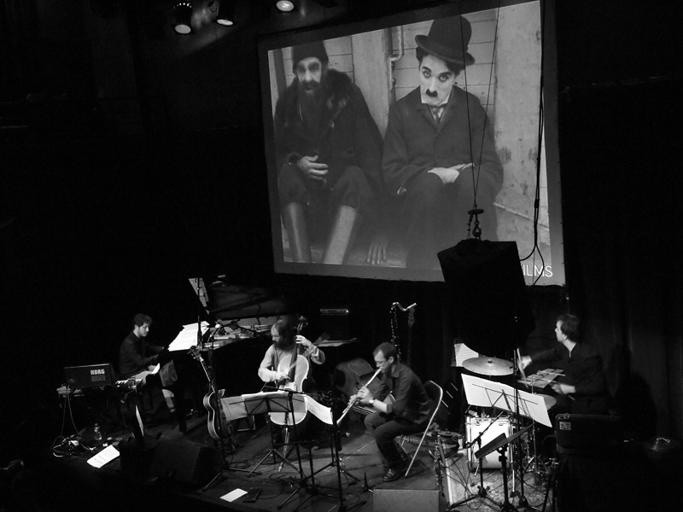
[368,397,376,406]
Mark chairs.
[397,374,448,481]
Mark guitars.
[189,346,230,442]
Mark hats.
[414,15,476,65]
[292,42,328,71]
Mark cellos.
[267,314,311,430]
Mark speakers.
[372,488,448,512]
[120,436,214,492]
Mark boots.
[321,205,363,265]
[283,202,313,263]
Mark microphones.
[209,323,221,338]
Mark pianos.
[165,316,209,354]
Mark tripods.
[449,353,556,512]
[201,337,373,512]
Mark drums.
[465,418,514,472]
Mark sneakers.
[383,456,411,482]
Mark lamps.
[172,2,192,37]
[215,1,237,31]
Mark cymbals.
[463,357,516,377]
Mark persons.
[347,342,438,482]
[514,313,610,461]
[380,14,504,268]
[117,313,205,427]
[256,319,326,450]
[271,35,383,265]
